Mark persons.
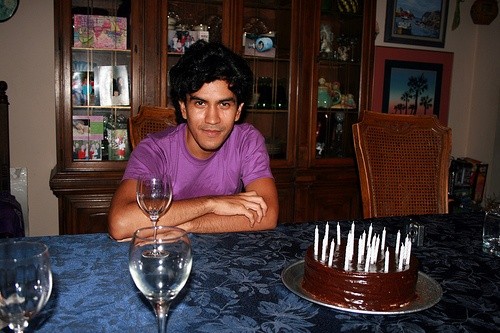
[73,121,90,134]
[106,73,129,105]
[117,141,126,160]
[81,79,93,105]
[172,33,196,53]
[108,41,279,246]
[73,142,101,160]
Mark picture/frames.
[384,0,450,47]
[371,45,455,128]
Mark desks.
[0,207,500,333]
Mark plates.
[281,259,444,315]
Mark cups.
[482,211,500,257]
[317,78,354,109]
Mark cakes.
[303,237,420,311]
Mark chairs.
[350,110,451,219]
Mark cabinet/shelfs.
[48,0,378,235]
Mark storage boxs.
[74,14,127,50]
[73,114,129,160]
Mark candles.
[314,221,412,272]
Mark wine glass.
[135,173,173,259]
[128,226,193,333]
[0,240,53,333]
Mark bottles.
[314,122,329,159]
